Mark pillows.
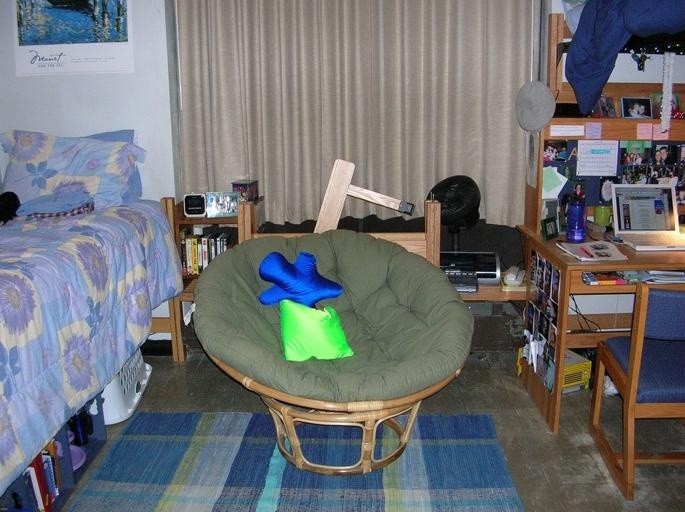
[81,128,141,201]
[280,299,354,363]
[1,131,146,206]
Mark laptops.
[611,184,685,251]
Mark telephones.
[503,266,526,286]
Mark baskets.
[88,347,153,425]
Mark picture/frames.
[620,96,655,120]
[541,216,558,240]
[205,190,240,219]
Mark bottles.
[566,195,588,242]
[71,406,90,447]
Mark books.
[180,228,230,276]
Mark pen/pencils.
[580,247,594,258]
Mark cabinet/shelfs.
[244,197,536,355]
[168,194,253,361]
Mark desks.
[513,0,685,434]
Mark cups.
[595,206,614,226]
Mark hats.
[515,79,556,131]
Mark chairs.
[197,229,474,476]
[590,280,684,498]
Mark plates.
[67,429,86,471]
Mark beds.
[1,125,183,503]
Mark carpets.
[59,411,527,510]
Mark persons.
[570,184,585,200]
[628,103,648,117]
[557,147,569,160]
[623,146,674,167]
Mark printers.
[439,250,500,292]
